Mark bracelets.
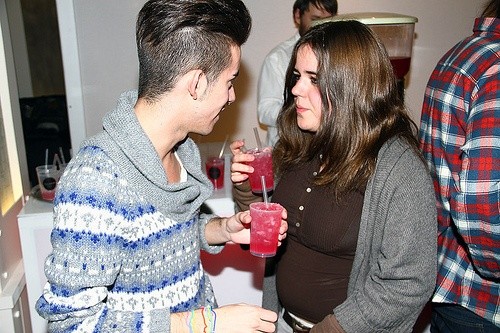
[174,305,217,333]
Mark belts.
[281,310,312,333]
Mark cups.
[206,159,225,190]
[244,147,275,193]
[36,163,67,200]
[249,202,284,257]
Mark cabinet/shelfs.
[17,141,264,333]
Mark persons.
[230,20,438,333]
[35,0,288,333]
[417,0,500,333]
[257,0,337,154]
